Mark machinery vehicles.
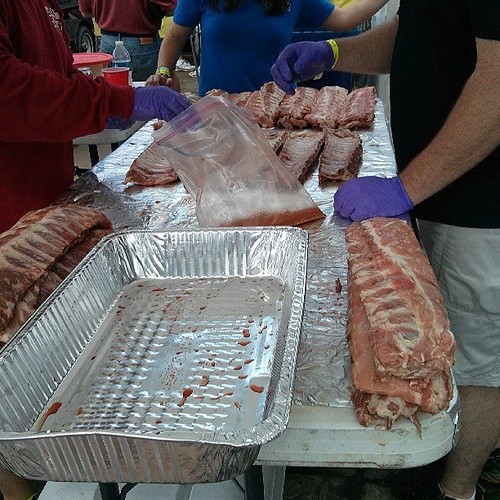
[59,0,102,55]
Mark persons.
[145,0,389,98]
[0,0,203,500]
[78,0,180,82]
[271,0,500,500]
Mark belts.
[101,29,156,38]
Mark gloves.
[103,116,136,131]
[334,176,414,223]
[129,85,205,135]
[270,38,338,96]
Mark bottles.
[113,41,133,85]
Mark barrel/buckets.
[73,53,112,86]
[101,67,130,85]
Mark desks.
[44,96,462,500]
[72,80,149,167]
